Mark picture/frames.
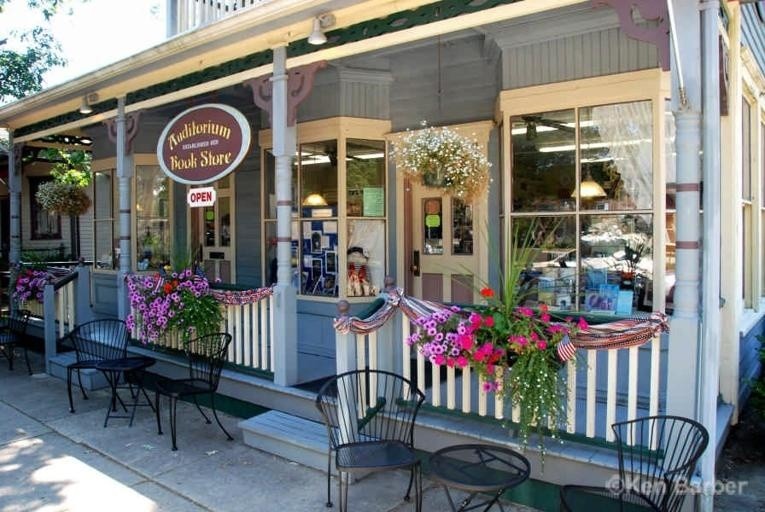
[310,230,337,280]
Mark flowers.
[35,181,91,217]
[406,212,593,475]
[124,250,227,360]
[388,119,495,207]
[8,249,59,304]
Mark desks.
[427,444,531,511]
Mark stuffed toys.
[347,246,372,297]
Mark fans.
[510,114,583,141]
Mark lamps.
[570,107,608,199]
[301,142,329,206]
[79,93,99,115]
[307,12,336,45]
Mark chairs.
[0,309,33,375]
[315,369,425,512]
[66,317,234,451]
[559,414,710,511]
